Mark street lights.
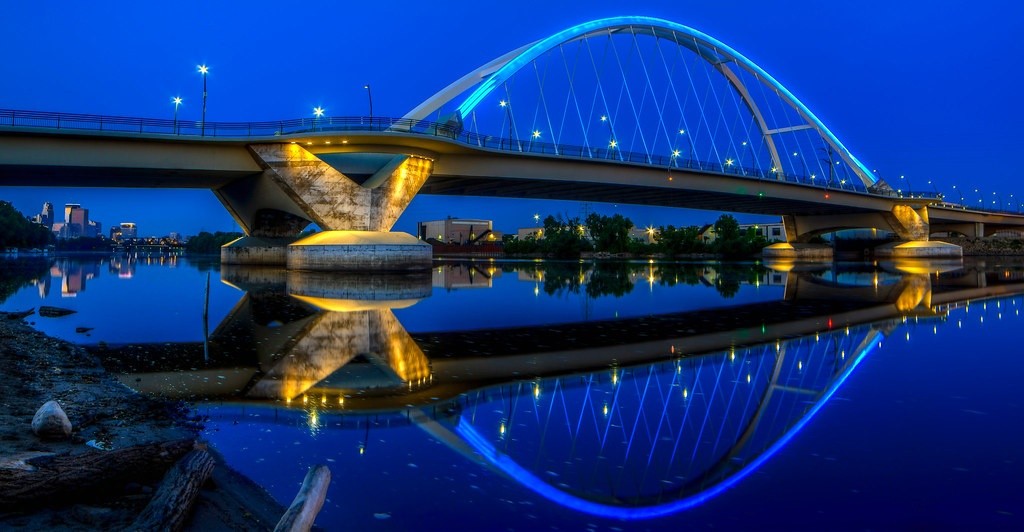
[992,191,1002,211]
[834,161,848,181]
[742,141,756,176]
[792,152,806,183]
[602,116,616,159]
[365,84,372,131]
[679,129,693,168]
[953,185,964,206]
[500,101,513,150]
[198,65,208,136]
[928,180,938,193]
[1010,194,1020,212]
[173,95,183,133]
[974,189,984,210]
[873,168,882,180]
[898,175,910,194]
